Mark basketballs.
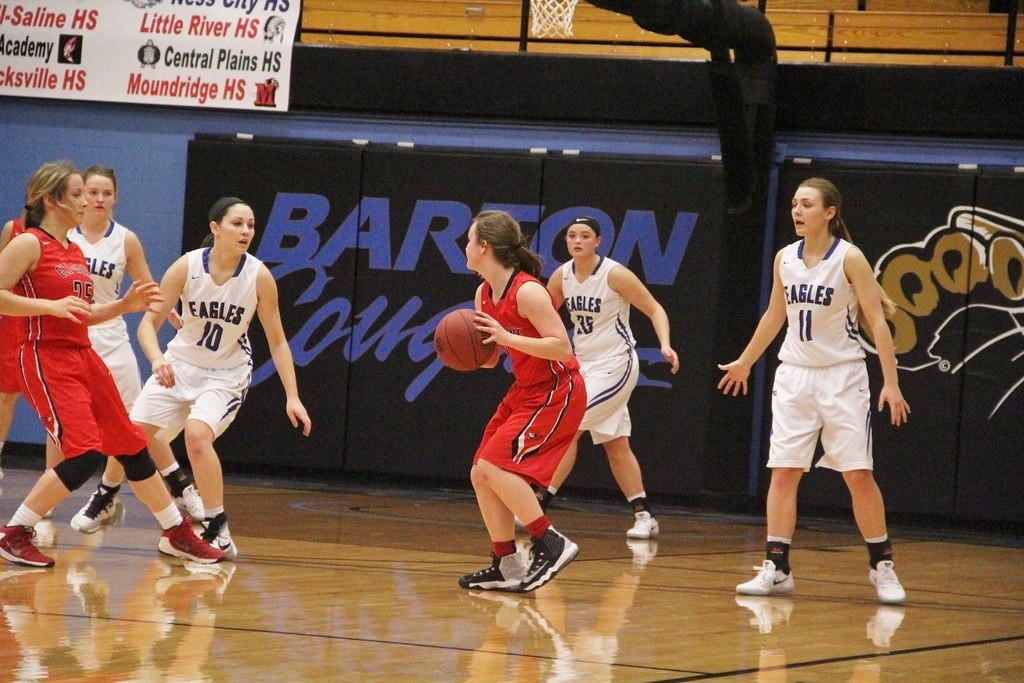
[433,308,497,371]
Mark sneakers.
[31,516,57,549]
[626,511,659,539]
[514,515,528,533]
[626,539,657,564]
[0,567,55,613]
[872,606,905,648]
[457,551,526,591]
[158,516,227,564]
[869,560,906,604]
[156,559,237,598]
[736,560,795,595]
[0,524,56,568]
[71,487,124,535]
[458,592,537,627]
[518,524,578,592]
[195,512,237,561]
[66,564,109,614]
[735,596,793,634]
[169,484,204,521]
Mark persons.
[719,178,912,602]
[0,158,228,569]
[0,566,232,683]
[734,596,905,683]
[70,197,312,563]
[515,217,680,540]
[458,211,588,592]
[466,539,660,683]
